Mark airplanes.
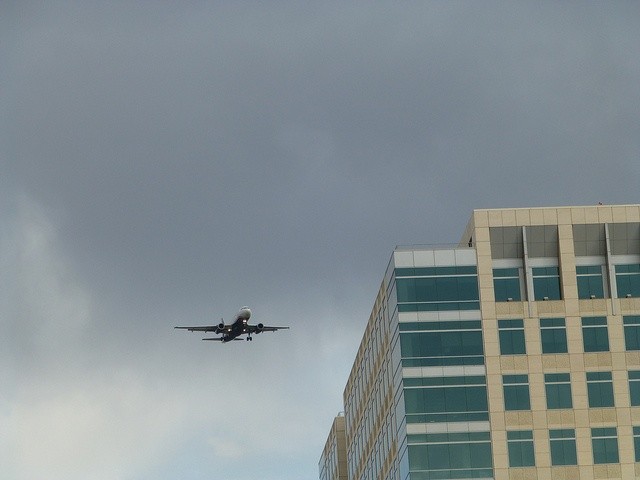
[175,306,290,344]
